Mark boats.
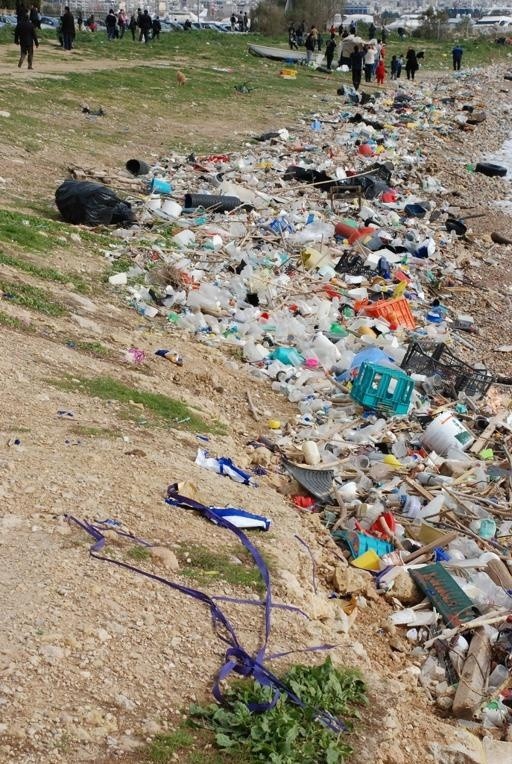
[246,41,325,63]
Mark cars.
[0,12,252,34]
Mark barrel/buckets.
[149,179,171,194]
[421,412,475,459]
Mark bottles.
[296,394,498,724]
[171,101,446,393]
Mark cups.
[143,304,160,318]
[107,272,129,287]
[489,664,505,687]
[150,178,171,196]
[148,198,181,219]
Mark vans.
[389,14,431,38]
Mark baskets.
[349,361,416,414]
[400,339,495,403]
[363,296,417,331]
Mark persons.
[452,45,463,71]
[237,10,244,31]
[1,13,6,22]
[185,18,192,29]
[230,13,236,31]
[288,18,419,89]
[173,18,178,26]
[12,0,162,69]
[244,11,249,30]
